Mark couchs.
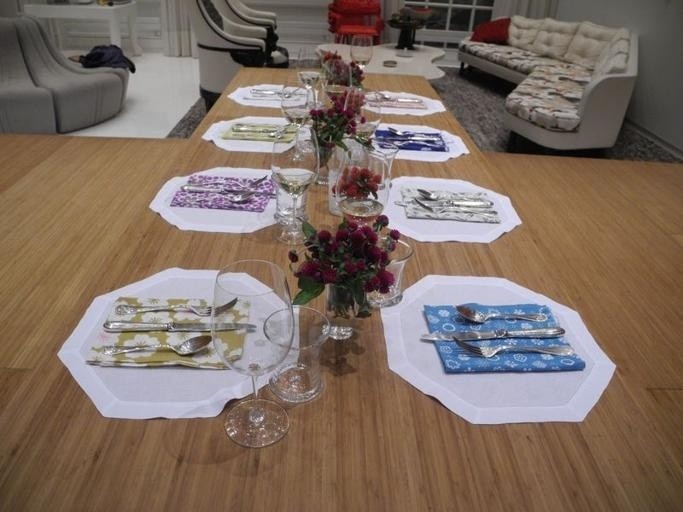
[456,14,640,152]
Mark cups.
[263,307,331,405]
[364,236,412,308]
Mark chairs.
[189,0,288,112]
[0,13,129,136]
[326,1,385,45]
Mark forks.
[453,337,574,356]
[115,297,236,318]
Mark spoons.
[101,335,211,356]
[454,305,549,325]
[365,90,500,225]
[180,87,308,208]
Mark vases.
[313,142,335,186]
[321,286,358,342]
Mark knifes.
[103,319,256,333]
[419,328,564,341]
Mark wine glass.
[271,32,401,243]
[210,258,292,449]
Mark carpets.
[164,66,678,159]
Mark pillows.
[471,18,509,43]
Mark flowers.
[332,166,384,203]
[308,86,366,163]
[320,49,365,85]
[287,213,402,319]
